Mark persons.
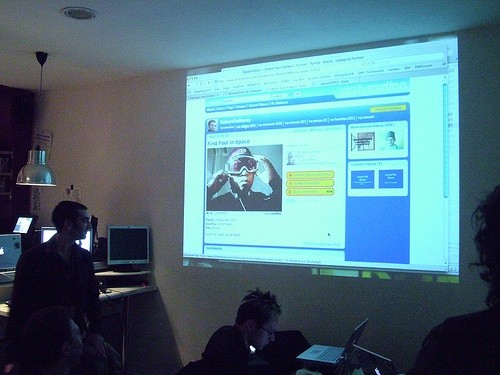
[202,287,281,375]
[207,147,282,211]
[208,120,217,133]
[405,186,500,375]
[4,201,120,375]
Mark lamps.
[15,50,58,187]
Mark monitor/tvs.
[0,234,22,271]
[41,227,93,258]
[108,226,150,272]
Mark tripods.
[12,214,39,245]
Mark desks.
[0,285,159,375]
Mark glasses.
[252,323,277,338]
[225,154,258,175]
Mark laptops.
[296,317,369,368]
